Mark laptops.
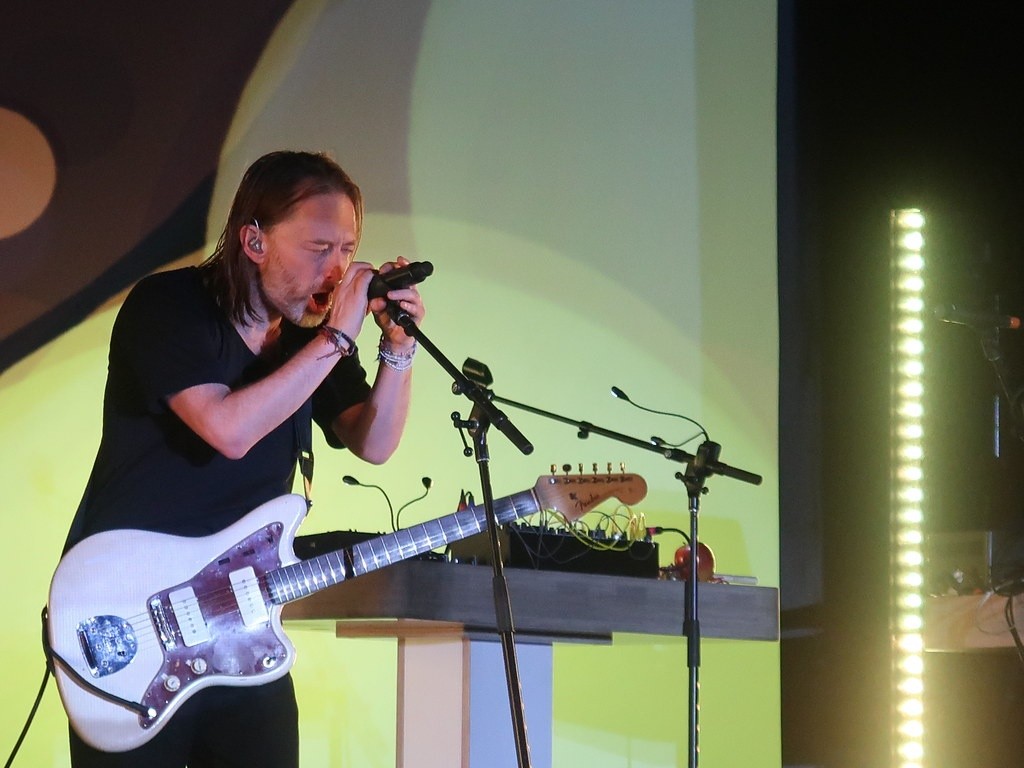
[926,531,996,596]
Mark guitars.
[48,462,648,753]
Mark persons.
[61,151,424,768]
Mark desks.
[280,560,779,768]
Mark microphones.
[367,261,435,301]
[934,305,1020,329]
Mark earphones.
[247,237,262,254]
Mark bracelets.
[321,325,358,360]
[379,335,417,372]
